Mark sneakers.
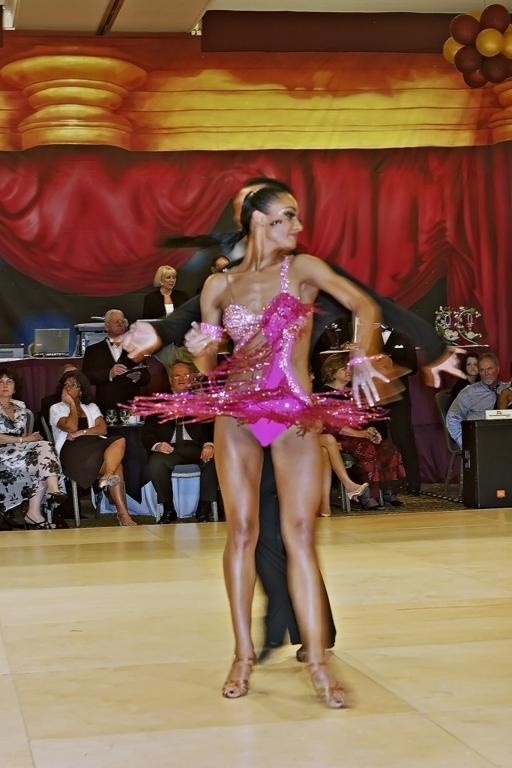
[254,632,335,665]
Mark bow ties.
[380,326,394,332]
[108,337,122,347]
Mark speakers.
[461,419,512,508]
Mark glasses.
[0,378,15,385]
[63,383,81,390]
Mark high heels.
[348,482,369,501]
[45,490,71,513]
[97,474,120,489]
[360,495,387,511]
[382,493,405,507]
[321,514,332,517]
[116,512,138,527]
[23,514,57,530]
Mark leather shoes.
[196,510,209,523]
[157,510,178,524]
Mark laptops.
[32,329,70,356]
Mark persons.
[118,186,389,707]
[447,353,481,414]
[111,176,466,662]
[496,386,512,410]
[446,353,512,450]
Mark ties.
[174,416,185,444]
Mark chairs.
[22,410,220,524]
[329,448,355,512]
[434,389,464,497]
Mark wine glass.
[106,409,117,425]
[120,409,130,425]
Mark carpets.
[420,486,464,503]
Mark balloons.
[442,4,512,89]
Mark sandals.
[222,650,257,699]
[305,664,352,708]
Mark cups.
[129,415,140,423]
